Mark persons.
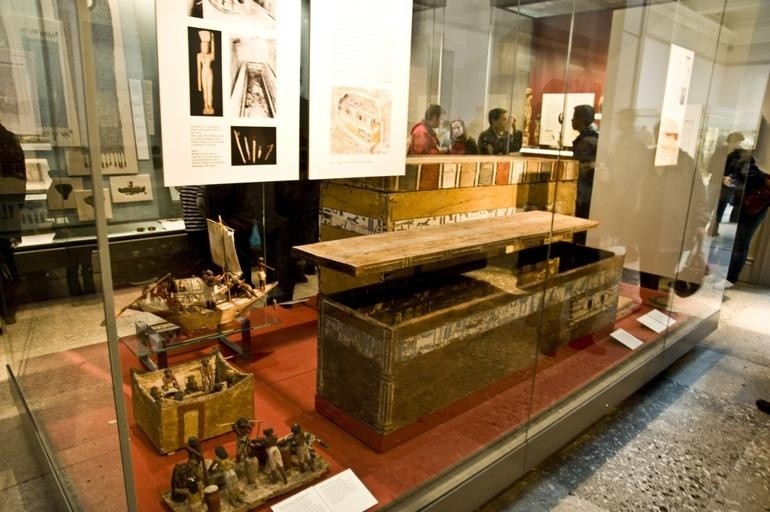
[174,415,318,512]
[181,79,320,311]
[46,144,109,305]
[478,107,522,155]
[150,357,214,399]
[406,104,450,154]
[0,123,27,325]
[607,104,747,313]
[442,120,479,154]
[572,104,599,217]
[707,115,770,290]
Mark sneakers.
[5,311,15,325]
[714,278,734,289]
[72,291,96,301]
